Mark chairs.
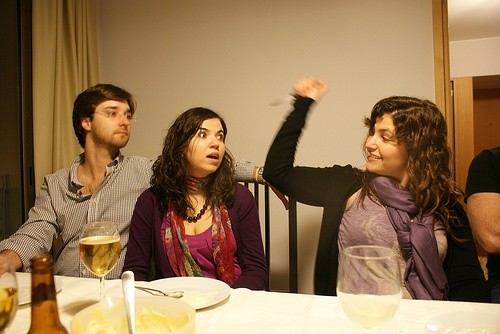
[237,180,298,293]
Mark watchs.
[257,166,266,185]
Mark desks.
[2,271,500,334]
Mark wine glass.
[336,245,403,333]
[78,221,121,303]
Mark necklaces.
[173,190,211,222]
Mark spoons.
[135,286,184,299]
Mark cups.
[68,294,196,334]
[0,257,19,334]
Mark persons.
[260,77,492,307]
[120,106,268,293]
[0,81,291,283]
[464,145,500,305]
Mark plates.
[18,284,62,306]
[138,276,231,309]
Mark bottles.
[26,255,68,334]
